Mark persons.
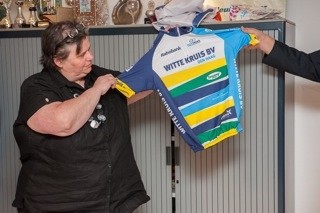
[241,24,320,85]
[12,21,158,213]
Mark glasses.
[53,22,88,45]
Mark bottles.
[0,0,38,28]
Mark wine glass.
[127,0,139,24]
